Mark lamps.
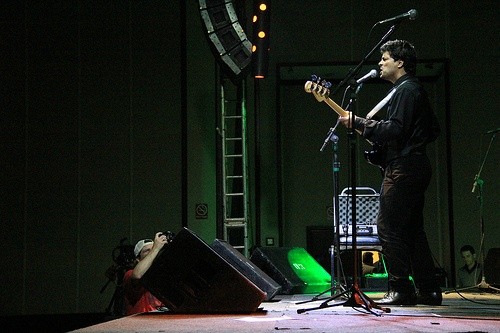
[252,0,272,79]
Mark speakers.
[209,238,282,302]
[141,227,267,314]
[248,247,308,296]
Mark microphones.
[488,127,500,134]
[347,69,378,87]
[379,9,417,24]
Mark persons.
[362,251,384,275]
[338,39,444,307]
[455,245,482,289]
[122,232,168,316]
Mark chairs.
[327,185,382,296]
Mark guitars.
[304,73,390,175]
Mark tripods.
[443,133,500,295]
[294,30,399,316]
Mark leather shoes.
[373,290,418,304]
[415,289,442,306]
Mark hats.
[134,239,154,257]
